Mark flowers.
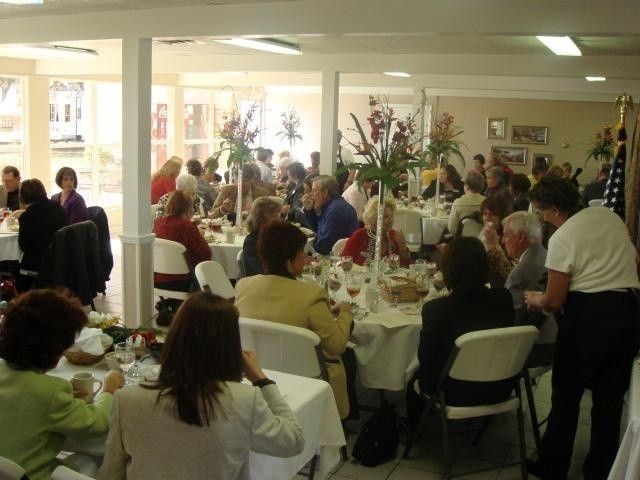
[209,101,269,177]
[276,109,302,147]
[332,94,432,204]
[583,123,615,170]
[423,111,469,169]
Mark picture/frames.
[486,118,548,165]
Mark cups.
[395,194,451,219]
[192,212,248,246]
[302,253,326,286]
[72,372,103,404]
[363,255,388,314]
[0,206,19,234]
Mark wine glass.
[299,198,313,214]
[389,281,403,309]
[115,341,133,389]
[388,254,400,276]
[330,253,341,273]
[340,255,352,278]
[405,256,448,314]
[347,278,362,308]
[127,338,144,379]
[327,274,343,302]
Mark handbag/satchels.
[352,406,398,466]
[155,296,184,325]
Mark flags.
[601,128,626,219]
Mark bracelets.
[253,378,278,386]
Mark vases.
[236,165,243,227]
[434,152,443,208]
[374,180,388,282]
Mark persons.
[336,129,356,195]
[525,171,639,480]
[96,292,305,479]
[422,154,612,276]
[234,219,362,436]
[501,211,561,368]
[151,149,356,292]
[343,167,411,265]
[407,235,516,444]
[0,166,90,295]
[1,285,125,480]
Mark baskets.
[65,349,106,365]
[378,276,421,302]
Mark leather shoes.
[527,459,566,480]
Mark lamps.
[537,35,583,57]
[221,38,303,54]
